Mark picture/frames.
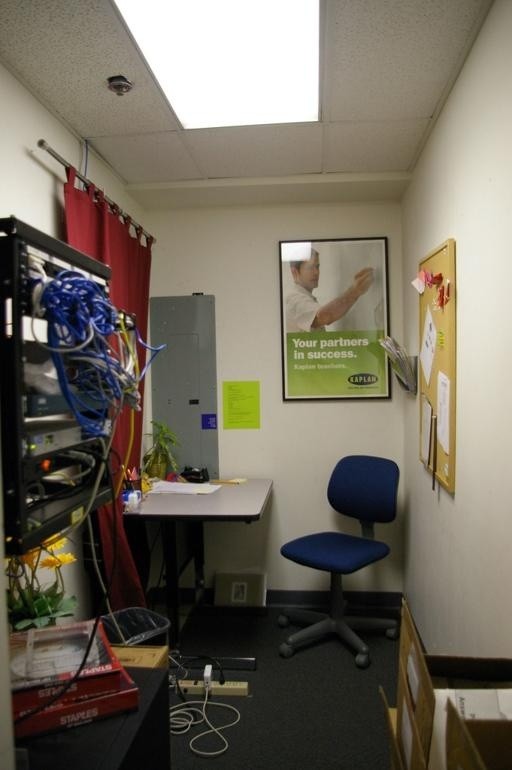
[276,235,393,402]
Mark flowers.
[7,529,78,628]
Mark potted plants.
[147,417,181,479]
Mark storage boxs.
[382,597,511,769]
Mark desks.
[125,474,271,669]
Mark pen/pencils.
[127,469,134,480]
[209,480,239,485]
[121,465,127,480]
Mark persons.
[286,245,375,333]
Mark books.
[393,344,416,390]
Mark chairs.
[277,450,400,665]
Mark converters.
[204,665,212,691]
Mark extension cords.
[176,680,248,697]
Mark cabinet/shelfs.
[4,641,172,768]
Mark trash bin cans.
[99,606,171,645]
